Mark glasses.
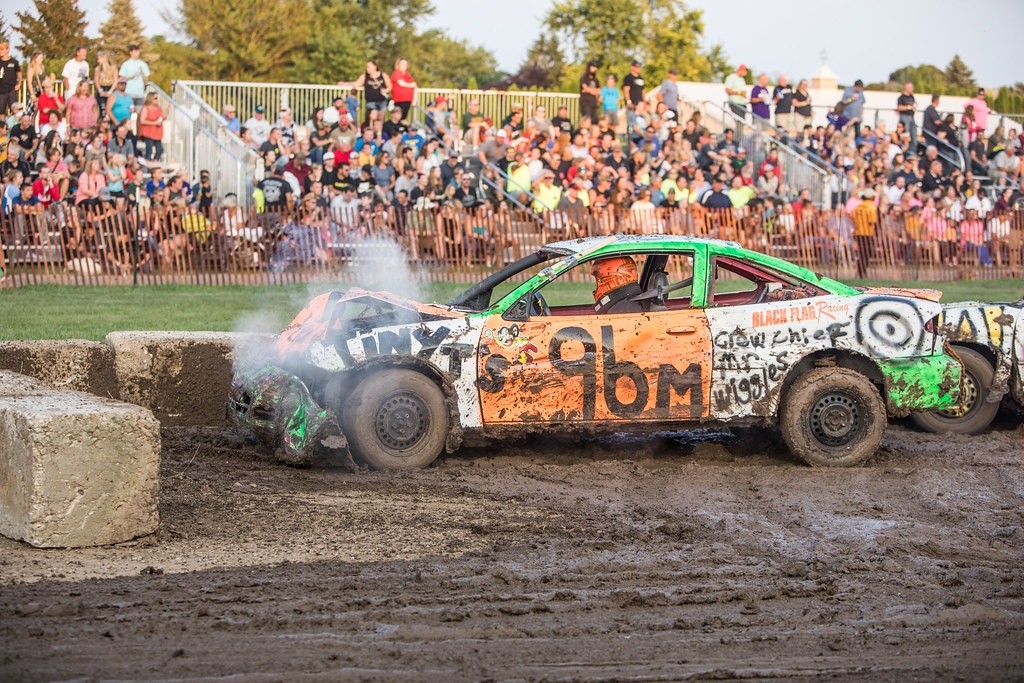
[154,97,158,99]
[120,81,126,85]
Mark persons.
[851,189,879,279]
[0,35,1024,274]
[591,256,649,314]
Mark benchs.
[770,233,817,263]
[505,211,544,258]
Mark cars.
[226,235,963,472]
[914,295,1019,435]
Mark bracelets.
[627,100,631,104]
[802,101,806,107]
[847,98,852,103]
[108,90,113,94]
[98,88,103,93]
[738,91,742,95]
[908,105,912,110]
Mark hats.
[222,196,237,207]
[765,166,774,170]
[543,170,555,177]
[255,105,265,113]
[569,182,584,191]
[324,153,333,159]
[738,64,747,71]
[497,129,506,136]
[403,164,415,172]
[302,192,315,207]
